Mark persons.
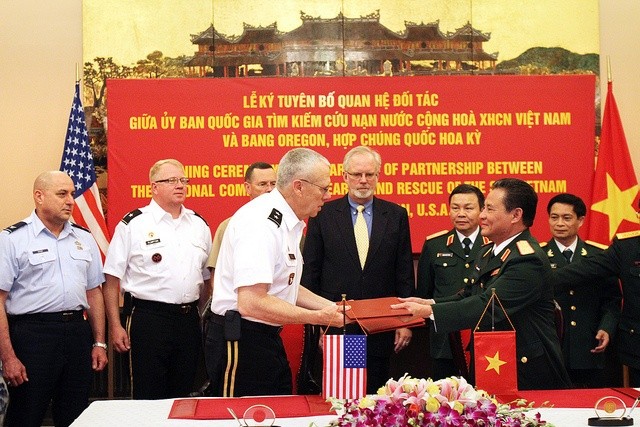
[299,146,416,394]
[417,183,492,386]
[205,161,277,290]
[552,193,622,387]
[101,158,214,400]
[554,199,640,386]
[1,170,109,427]
[390,177,574,389]
[206,148,356,398]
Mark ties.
[563,251,573,264]
[354,205,369,271]
[462,238,471,259]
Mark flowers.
[324,371,554,427]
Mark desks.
[67,386,640,427]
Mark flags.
[60,81,110,266]
[585,82,640,250]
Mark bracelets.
[93,342,108,350]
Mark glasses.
[344,170,380,180]
[300,179,332,195]
[155,177,190,185]
